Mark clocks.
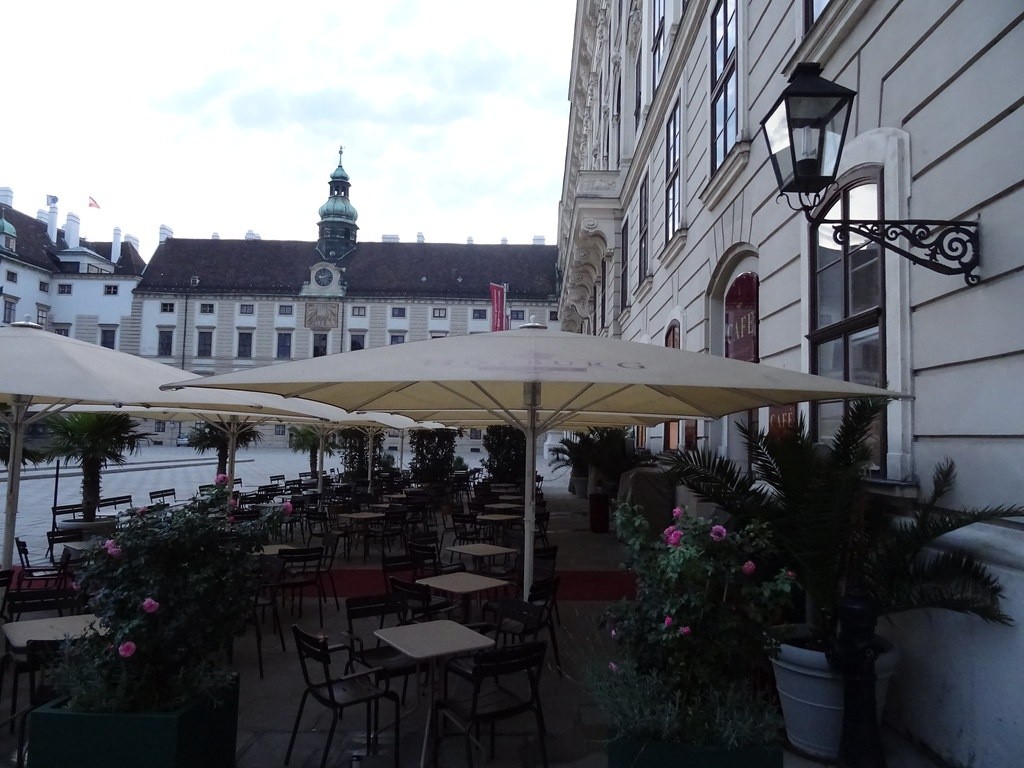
[315,268,333,286]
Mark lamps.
[760,61,982,287]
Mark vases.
[27,671,239,768]
[606,715,786,768]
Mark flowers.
[28,474,294,713]
[546,497,786,749]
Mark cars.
[176,434,191,448]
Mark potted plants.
[546,426,656,497]
[44,412,159,544]
[649,397,1024,761]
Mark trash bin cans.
[589,491,610,533]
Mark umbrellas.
[1,315,914,602]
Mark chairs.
[0,467,552,768]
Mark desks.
[484,503,524,508]
[415,572,510,623]
[370,620,488,768]
[337,511,387,556]
[368,504,403,508]
[490,483,516,486]
[490,489,519,494]
[403,488,426,493]
[383,494,407,498]
[2,614,110,734]
[250,504,284,509]
[62,541,102,551]
[476,514,523,521]
[445,544,519,573]
[330,482,350,487]
[249,545,297,557]
[494,495,523,501]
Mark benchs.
[149,489,176,506]
[198,478,242,496]
[52,495,133,540]
[269,475,285,483]
[299,472,311,481]
